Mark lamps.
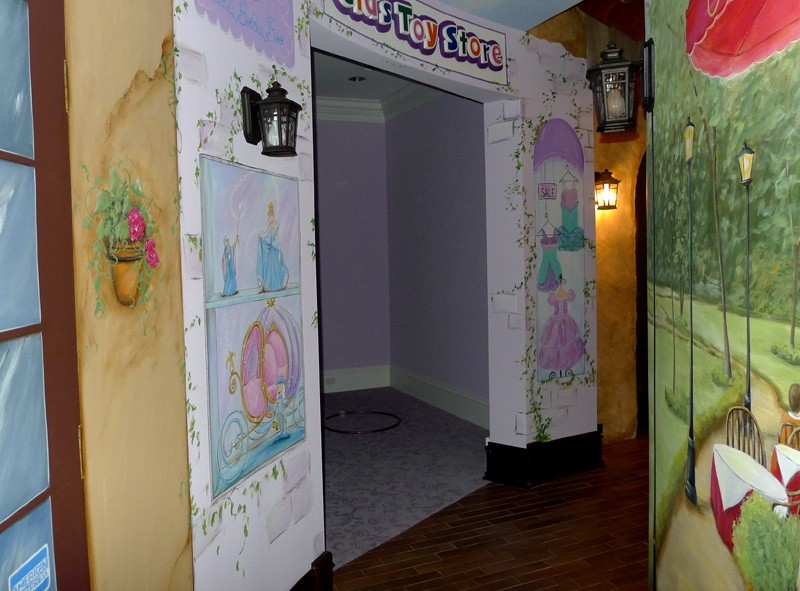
[594,168,621,209]
[584,36,655,132]
[239,79,303,159]
